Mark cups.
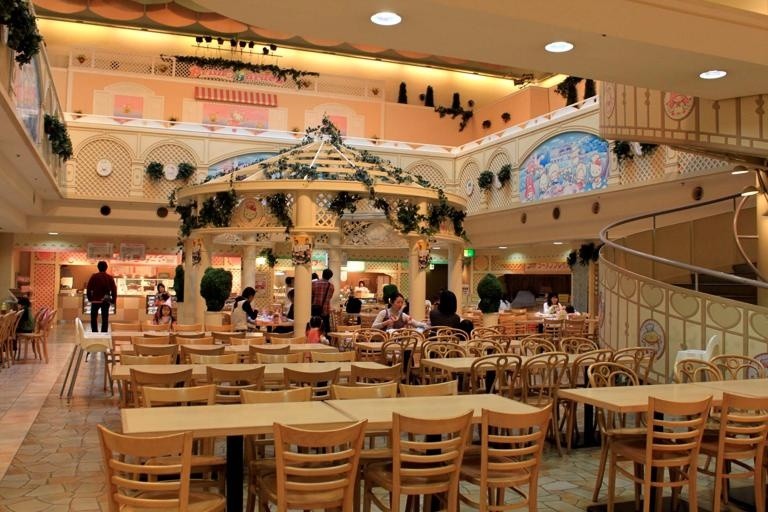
[274,313,280,323]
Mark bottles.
[282,312,287,322]
[1,303,7,314]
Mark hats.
[161,297,172,308]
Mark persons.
[152,303,177,333]
[541,291,562,331]
[370,292,428,382]
[230,286,259,332]
[12,297,34,351]
[564,304,581,320]
[154,283,172,319]
[86,260,117,333]
[285,276,294,314]
[304,314,331,386]
[272,288,294,334]
[353,280,369,294]
[499,292,511,309]
[428,289,474,342]
[312,267,334,346]
[312,272,319,281]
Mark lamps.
[740,187,760,199]
[731,166,749,178]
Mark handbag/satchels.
[311,304,324,316]
[103,295,111,304]
[345,298,361,313]
